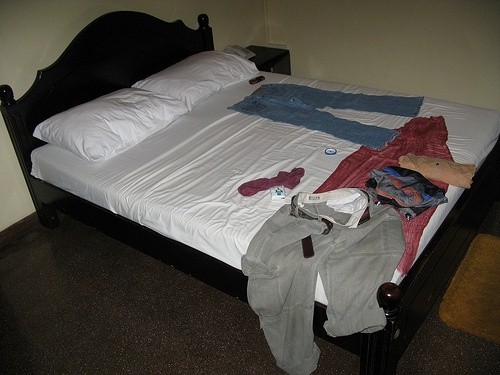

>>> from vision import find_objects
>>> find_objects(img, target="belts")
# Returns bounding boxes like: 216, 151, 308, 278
290, 187, 370, 258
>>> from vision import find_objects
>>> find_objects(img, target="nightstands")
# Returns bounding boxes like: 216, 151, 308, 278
244, 44, 292, 77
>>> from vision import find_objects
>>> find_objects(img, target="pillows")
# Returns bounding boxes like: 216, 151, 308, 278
32, 88, 191, 162
130, 50, 259, 113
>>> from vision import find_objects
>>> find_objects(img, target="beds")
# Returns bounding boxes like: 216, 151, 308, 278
0, 9, 500, 375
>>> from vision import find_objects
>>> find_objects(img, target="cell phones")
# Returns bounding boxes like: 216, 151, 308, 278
249, 75, 265, 84
